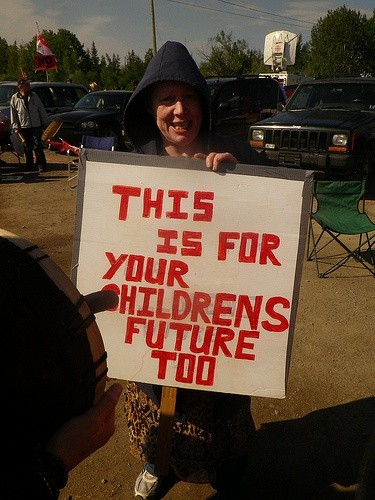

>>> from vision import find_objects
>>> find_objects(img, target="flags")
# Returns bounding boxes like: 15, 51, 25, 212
36, 30, 53, 55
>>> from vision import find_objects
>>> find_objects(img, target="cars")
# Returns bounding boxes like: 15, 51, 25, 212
46, 89, 138, 143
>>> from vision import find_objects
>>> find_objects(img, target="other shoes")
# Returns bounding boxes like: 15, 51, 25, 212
38, 163, 46, 171
135, 468, 161, 499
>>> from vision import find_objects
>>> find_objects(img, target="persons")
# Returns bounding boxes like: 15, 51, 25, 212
86, 82, 98, 103
121, 40, 259, 500
0, 290, 123, 500
10, 78, 50, 172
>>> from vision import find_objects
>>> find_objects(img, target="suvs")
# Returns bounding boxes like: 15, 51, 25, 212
246, 77, 375, 193
0, 82, 93, 138
198, 74, 286, 151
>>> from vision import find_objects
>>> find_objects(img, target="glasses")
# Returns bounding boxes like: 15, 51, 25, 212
159, 95, 199, 106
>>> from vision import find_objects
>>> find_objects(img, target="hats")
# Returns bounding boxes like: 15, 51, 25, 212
18, 77, 32, 84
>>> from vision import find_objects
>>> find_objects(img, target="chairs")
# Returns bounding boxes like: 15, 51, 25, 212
67, 136, 115, 186
307, 181, 375, 276
